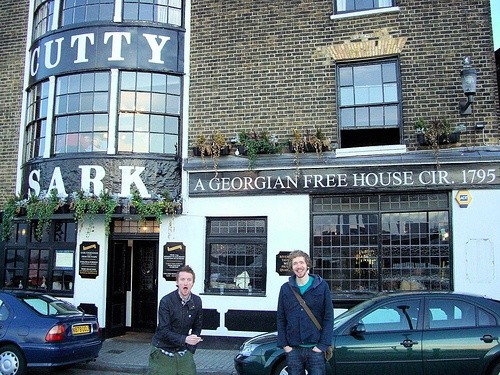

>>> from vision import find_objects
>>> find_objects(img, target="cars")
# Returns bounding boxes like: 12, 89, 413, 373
233, 290, 500, 375
0, 287, 105, 375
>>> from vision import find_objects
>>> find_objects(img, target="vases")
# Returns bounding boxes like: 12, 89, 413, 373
193, 146, 229, 156
416, 132, 460, 146
289, 142, 329, 153
237, 144, 267, 155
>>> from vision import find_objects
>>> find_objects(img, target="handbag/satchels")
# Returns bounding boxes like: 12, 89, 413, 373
324, 343, 335, 363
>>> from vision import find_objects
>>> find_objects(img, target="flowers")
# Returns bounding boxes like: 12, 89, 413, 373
1, 188, 182, 241
234, 131, 279, 173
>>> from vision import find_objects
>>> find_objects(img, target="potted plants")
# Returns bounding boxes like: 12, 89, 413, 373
192, 131, 229, 177
287, 128, 331, 172
413, 120, 463, 169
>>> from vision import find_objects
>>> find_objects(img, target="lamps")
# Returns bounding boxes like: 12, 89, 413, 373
459, 56, 477, 116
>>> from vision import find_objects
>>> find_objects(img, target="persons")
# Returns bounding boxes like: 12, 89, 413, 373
333, 281, 343, 290
79, 133, 132, 153
277, 249, 334, 375
148, 266, 203, 375
401, 268, 428, 291
